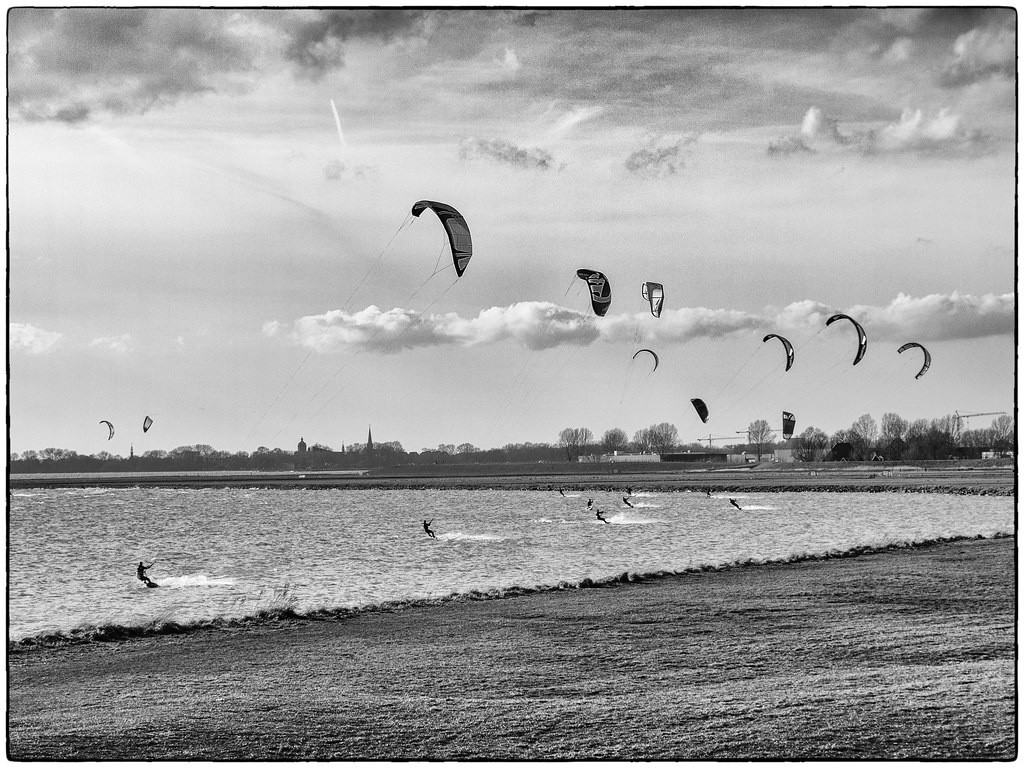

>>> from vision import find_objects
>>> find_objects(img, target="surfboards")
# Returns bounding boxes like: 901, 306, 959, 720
146, 582, 160, 589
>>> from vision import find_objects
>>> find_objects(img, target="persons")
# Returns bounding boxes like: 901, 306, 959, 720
707, 486, 712, 497
137, 562, 151, 584
588, 499, 593, 510
596, 509, 608, 523
627, 487, 633, 495
623, 497, 632, 507
730, 498, 741, 509
423, 520, 435, 538
560, 488, 564, 496
